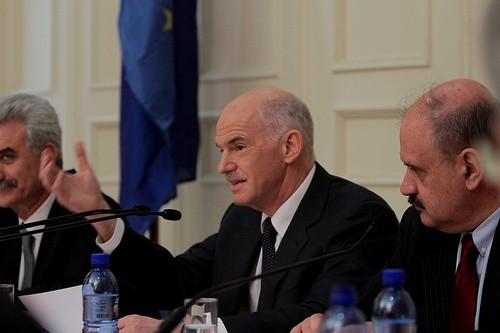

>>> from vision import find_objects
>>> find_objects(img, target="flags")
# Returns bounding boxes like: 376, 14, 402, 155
118, 0, 200, 236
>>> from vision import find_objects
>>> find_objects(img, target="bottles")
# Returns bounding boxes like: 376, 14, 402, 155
372, 269, 416, 333
82, 254, 120, 333
317, 285, 367, 333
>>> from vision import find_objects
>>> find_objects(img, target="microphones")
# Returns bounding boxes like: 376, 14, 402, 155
1, 205, 150, 233
154, 208, 385, 333
0, 209, 182, 242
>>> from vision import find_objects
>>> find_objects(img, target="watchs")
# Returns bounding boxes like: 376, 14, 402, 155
181, 324, 196, 333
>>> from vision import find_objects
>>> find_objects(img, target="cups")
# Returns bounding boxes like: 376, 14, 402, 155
184, 297, 218, 333
0, 284, 14, 303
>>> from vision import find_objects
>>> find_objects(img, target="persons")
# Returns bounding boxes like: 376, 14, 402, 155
38, 86, 402, 333
290, 78, 500, 333
0, 93, 128, 310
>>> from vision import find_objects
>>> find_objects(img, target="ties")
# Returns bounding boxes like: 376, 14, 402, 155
254, 217, 276, 290
447, 233, 479, 333
21, 222, 36, 294
